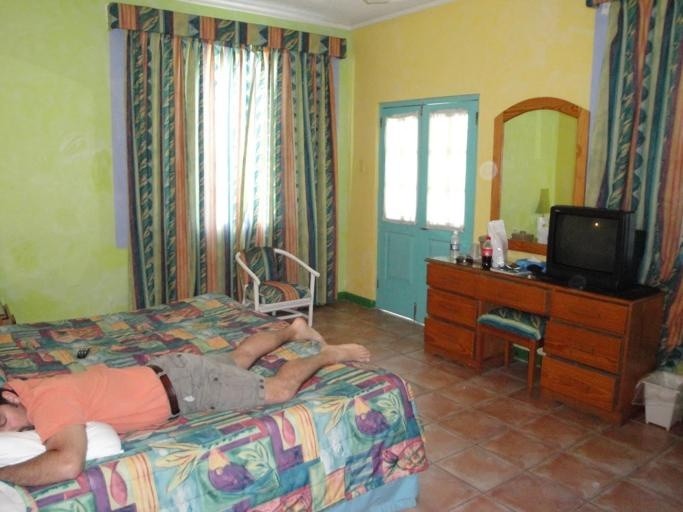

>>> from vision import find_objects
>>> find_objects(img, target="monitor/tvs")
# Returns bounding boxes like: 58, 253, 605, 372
548, 204, 648, 295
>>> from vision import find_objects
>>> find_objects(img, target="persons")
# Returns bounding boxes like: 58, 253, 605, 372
1, 316, 373, 488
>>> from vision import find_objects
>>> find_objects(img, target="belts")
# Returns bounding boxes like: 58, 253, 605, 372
147, 364, 181, 420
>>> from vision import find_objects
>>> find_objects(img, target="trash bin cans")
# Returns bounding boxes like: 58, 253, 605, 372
641, 370, 683, 432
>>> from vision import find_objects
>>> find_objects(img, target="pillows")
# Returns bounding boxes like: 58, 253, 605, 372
1, 422, 124, 468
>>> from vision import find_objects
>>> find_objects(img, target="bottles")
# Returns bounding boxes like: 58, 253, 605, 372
449, 230, 461, 262
481, 235, 494, 270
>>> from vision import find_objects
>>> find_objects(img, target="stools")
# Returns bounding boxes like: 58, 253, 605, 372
476, 307, 547, 396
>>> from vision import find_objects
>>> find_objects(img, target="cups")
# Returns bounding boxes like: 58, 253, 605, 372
469, 244, 480, 258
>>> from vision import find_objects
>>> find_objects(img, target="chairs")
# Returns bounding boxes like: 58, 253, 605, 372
235, 246, 321, 328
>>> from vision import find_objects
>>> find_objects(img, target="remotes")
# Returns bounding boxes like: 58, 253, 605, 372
77, 345, 91, 359
455, 254, 474, 265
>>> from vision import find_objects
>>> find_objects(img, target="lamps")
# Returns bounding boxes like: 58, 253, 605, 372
535, 188, 551, 244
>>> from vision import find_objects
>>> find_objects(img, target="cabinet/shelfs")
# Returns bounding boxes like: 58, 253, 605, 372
423, 251, 669, 426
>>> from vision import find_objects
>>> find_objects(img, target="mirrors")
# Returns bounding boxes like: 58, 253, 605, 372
489, 96, 591, 256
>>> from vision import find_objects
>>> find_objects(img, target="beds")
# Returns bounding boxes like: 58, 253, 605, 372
0, 292, 416, 512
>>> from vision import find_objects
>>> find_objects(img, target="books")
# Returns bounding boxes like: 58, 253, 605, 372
490, 264, 534, 275
